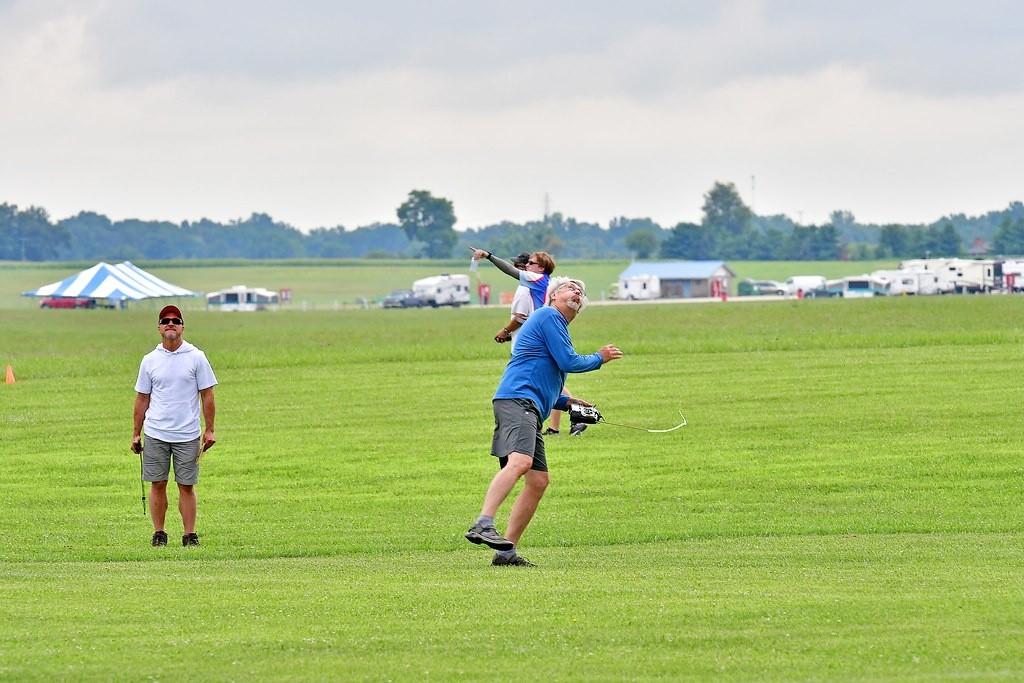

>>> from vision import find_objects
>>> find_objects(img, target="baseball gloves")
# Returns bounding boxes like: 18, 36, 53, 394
568, 404, 607, 436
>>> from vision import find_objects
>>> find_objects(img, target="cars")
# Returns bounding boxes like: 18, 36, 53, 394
804, 285, 843, 299
382, 288, 424, 309
754, 279, 787, 296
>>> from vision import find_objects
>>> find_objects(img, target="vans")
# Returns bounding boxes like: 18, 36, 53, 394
783, 276, 827, 295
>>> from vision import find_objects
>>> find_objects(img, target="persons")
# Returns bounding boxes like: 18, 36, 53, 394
464, 275, 624, 566
510, 253, 530, 271
469, 245, 588, 436
131, 305, 216, 547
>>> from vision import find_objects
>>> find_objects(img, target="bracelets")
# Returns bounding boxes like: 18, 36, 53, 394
504, 327, 510, 333
486, 251, 492, 259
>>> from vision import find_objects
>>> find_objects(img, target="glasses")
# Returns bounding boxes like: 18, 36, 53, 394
557, 283, 586, 297
525, 261, 539, 265
514, 263, 525, 268
159, 318, 182, 325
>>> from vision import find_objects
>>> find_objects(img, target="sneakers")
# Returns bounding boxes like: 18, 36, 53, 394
464, 523, 514, 551
181, 533, 201, 547
150, 531, 168, 547
569, 422, 587, 436
492, 553, 537, 567
541, 426, 559, 435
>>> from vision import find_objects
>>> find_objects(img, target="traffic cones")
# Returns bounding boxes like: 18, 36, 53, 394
4, 365, 17, 384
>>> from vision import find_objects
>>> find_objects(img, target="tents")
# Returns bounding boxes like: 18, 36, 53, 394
20, 261, 203, 312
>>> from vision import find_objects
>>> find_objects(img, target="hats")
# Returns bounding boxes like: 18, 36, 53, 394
510, 253, 530, 266
158, 305, 184, 325
